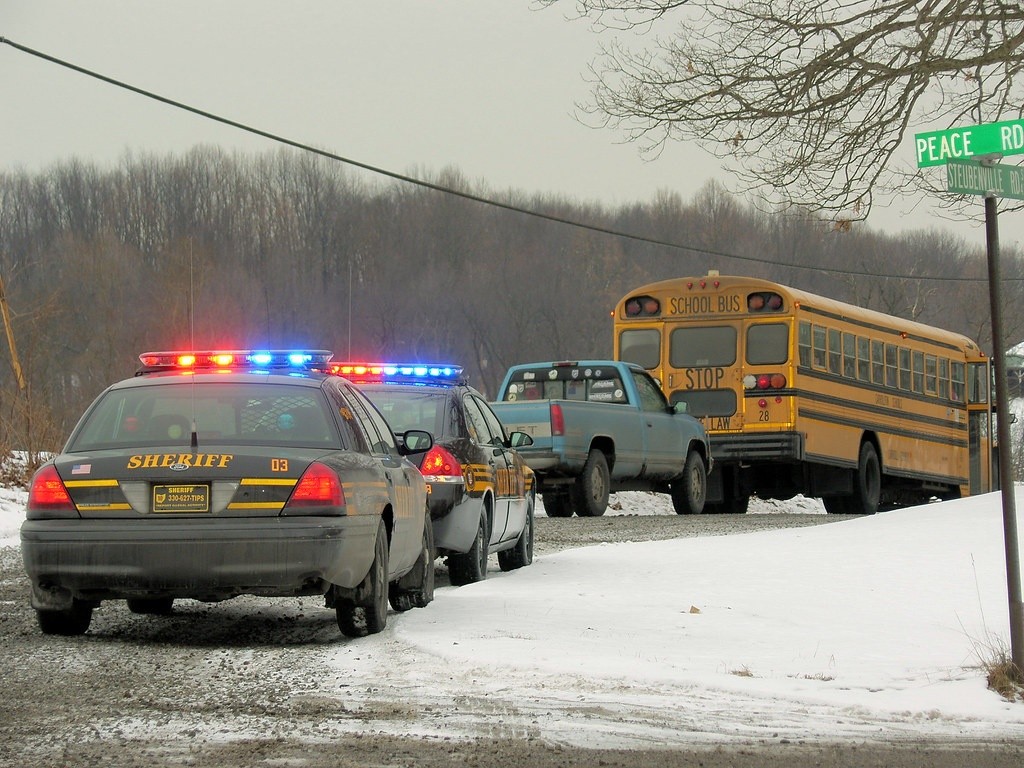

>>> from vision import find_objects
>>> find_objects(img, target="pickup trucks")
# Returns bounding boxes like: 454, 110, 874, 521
481, 357, 713, 516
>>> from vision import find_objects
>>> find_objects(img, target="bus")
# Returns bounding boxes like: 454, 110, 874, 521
611, 269, 995, 517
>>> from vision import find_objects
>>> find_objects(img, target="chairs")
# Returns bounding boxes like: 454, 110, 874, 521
149, 413, 191, 441
286, 407, 330, 442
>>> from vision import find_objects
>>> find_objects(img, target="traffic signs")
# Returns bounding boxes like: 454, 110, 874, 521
943, 157, 1024, 201
912, 115, 1024, 169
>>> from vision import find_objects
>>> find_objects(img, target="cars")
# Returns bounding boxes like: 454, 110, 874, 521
17, 346, 440, 639
310, 360, 538, 587
